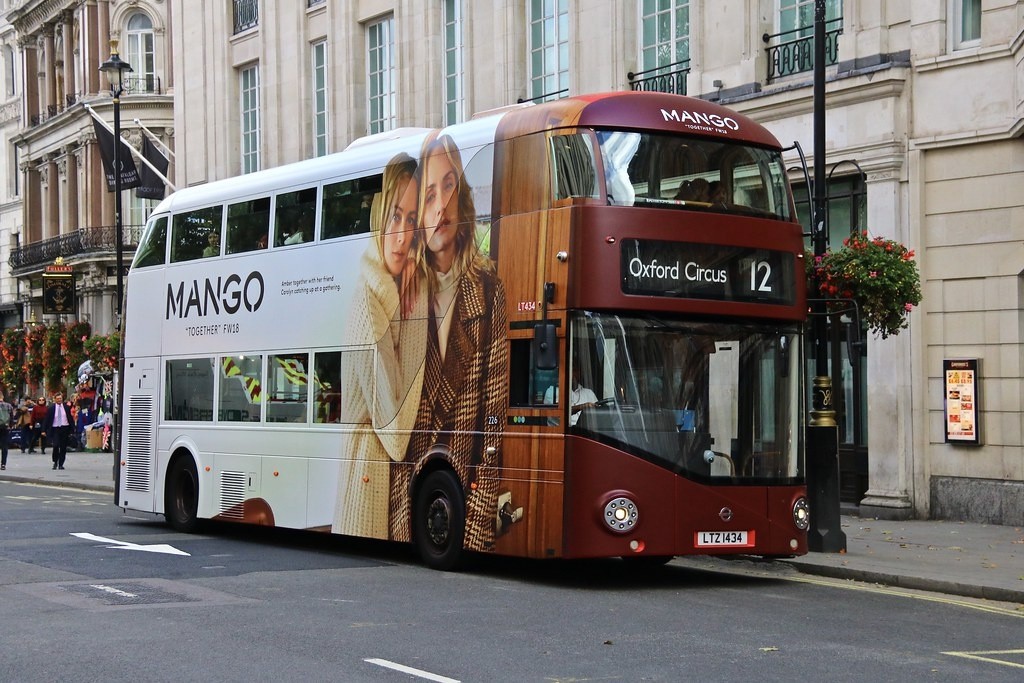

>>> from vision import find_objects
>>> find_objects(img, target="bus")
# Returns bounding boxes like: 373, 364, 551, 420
113, 91, 811, 571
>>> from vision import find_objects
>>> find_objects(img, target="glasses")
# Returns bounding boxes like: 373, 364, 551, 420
38, 399, 45, 401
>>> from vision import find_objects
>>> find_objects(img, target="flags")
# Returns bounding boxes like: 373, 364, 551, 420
91, 113, 142, 193
134, 132, 170, 200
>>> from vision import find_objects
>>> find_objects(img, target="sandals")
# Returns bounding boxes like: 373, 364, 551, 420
1, 464, 5, 470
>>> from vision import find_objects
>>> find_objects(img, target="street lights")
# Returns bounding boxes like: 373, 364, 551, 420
97, 39, 135, 329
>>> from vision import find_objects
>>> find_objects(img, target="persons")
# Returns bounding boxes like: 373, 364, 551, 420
329, 146, 432, 540
173, 190, 374, 423
40, 391, 77, 470
3, 395, 79, 455
543, 360, 599, 426
0, 390, 14, 470
385, 131, 509, 552
672, 178, 729, 205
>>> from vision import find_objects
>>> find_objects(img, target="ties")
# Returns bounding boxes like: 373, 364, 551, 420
58, 405, 62, 422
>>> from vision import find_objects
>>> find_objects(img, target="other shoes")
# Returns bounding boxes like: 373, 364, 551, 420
28, 450, 37, 454
21, 450, 25, 453
52, 461, 57, 470
41, 448, 46, 454
59, 464, 64, 469
